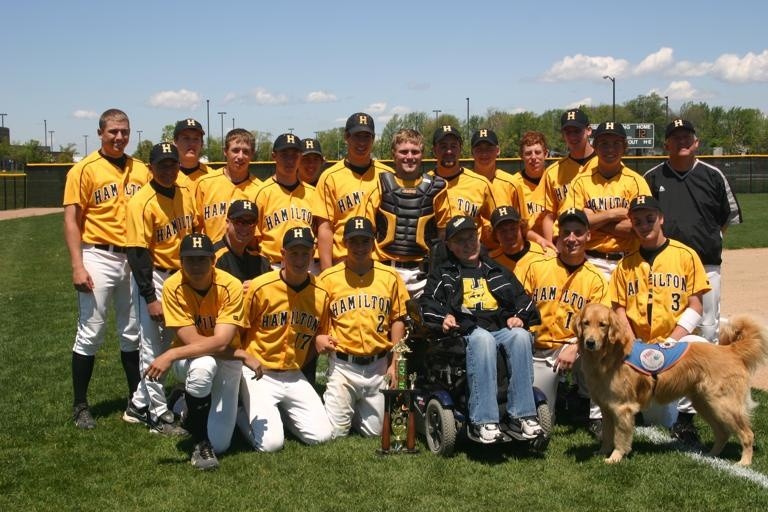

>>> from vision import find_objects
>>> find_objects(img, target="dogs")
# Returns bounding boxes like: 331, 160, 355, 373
572, 302, 768, 468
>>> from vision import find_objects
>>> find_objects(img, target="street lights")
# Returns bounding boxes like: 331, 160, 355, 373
136, 129, 144, 142
217, 111, 228, 147
663, 95, 669, 122
335, 128, 342, 161
415, 114, 420, 128
43, 119, 49, 147
313, 131, 319, 140
465, 97, 471, 141
288, 128, 295, 134
432, 109, 443, 128
47, 129, 55, 152
206, 99, 211, 145
0, 114, 9, 128
602, 75, 617, 124
82, 134, 89, 157
232, 116, 235, 130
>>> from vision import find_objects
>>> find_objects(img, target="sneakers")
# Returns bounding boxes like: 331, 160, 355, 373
672, 423, 708, 456
475, 414, 548, 444
72, 403, 98, 432
123, 403, 220, 473
588, 415, 605, 439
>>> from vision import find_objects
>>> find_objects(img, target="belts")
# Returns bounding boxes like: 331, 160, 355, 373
94, 245, 128, 254
334, 352, 386, 367
384, 260, 422, 269
154, 264, 178, 274
586, 250, 625, 261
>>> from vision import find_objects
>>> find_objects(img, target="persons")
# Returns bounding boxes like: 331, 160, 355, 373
610, 196, 712, 448
62, 109, 154, 431
122, 112, 651, 470
642, 120, 741, 337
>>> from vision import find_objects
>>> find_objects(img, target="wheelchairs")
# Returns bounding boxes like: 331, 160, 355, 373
405, 292, 554, 459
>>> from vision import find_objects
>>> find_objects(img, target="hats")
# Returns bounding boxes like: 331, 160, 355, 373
557, 109, 695, 227
431, 125, 520, 240
148, 112, 376, 257
